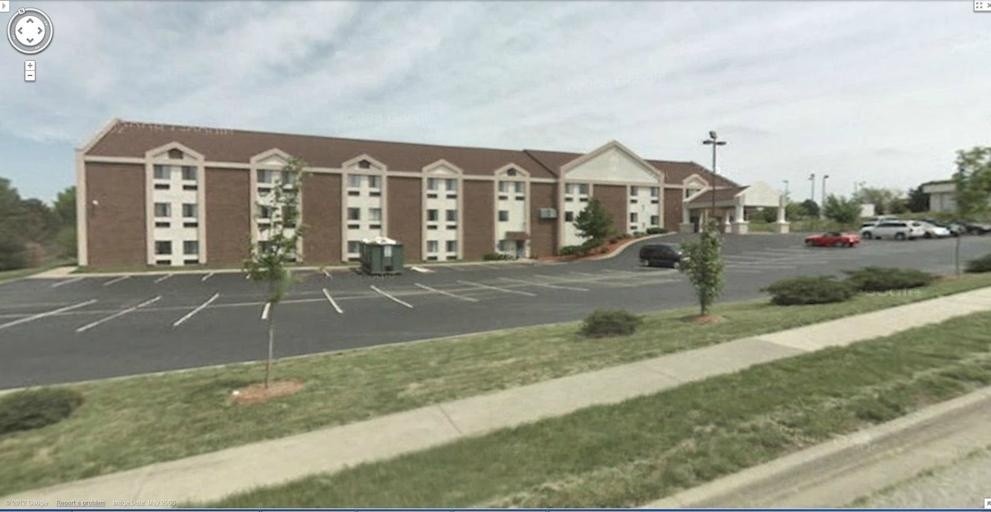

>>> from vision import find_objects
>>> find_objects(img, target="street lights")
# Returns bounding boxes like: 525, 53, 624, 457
822, 172, 829, 203
808, 171, 816, 201
703, 129, 727, 214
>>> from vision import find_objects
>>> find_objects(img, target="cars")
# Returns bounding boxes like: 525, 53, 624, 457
859, 215, 991, 241
802, 231, 860, 248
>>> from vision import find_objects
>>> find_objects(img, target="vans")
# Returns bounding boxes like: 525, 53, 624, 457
639, 243, 691, 269
